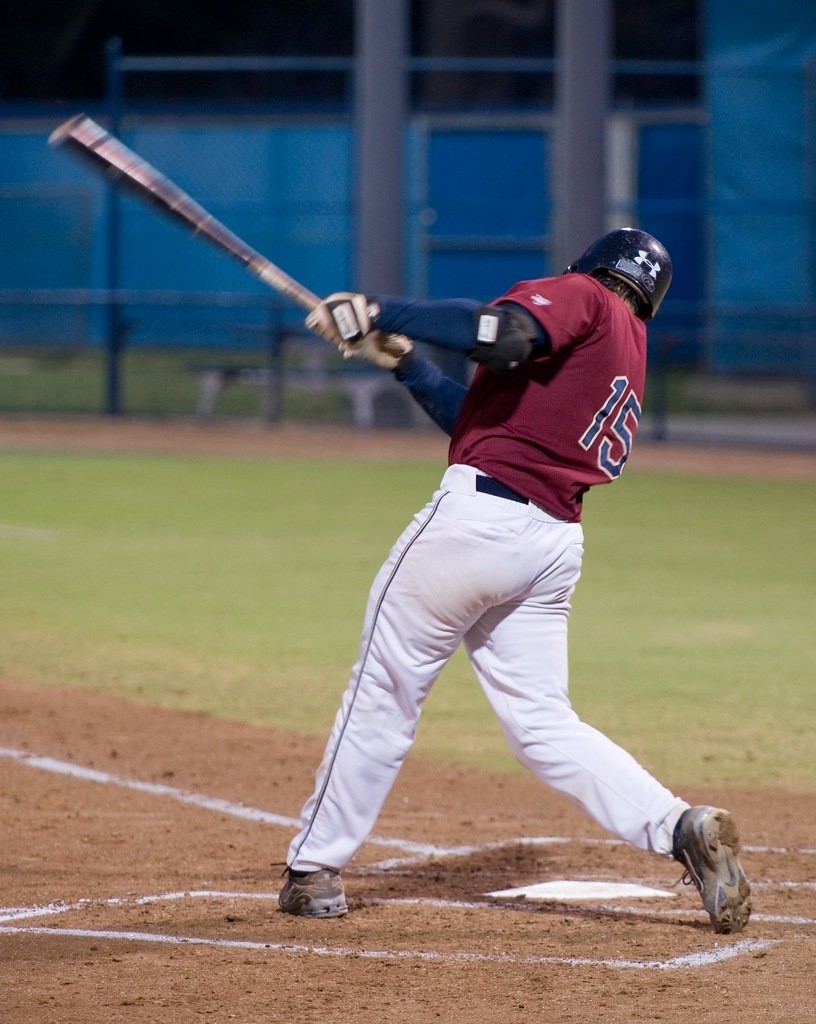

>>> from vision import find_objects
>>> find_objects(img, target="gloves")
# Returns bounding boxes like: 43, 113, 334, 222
342, 328, 413, 371
303, 291, 378, 351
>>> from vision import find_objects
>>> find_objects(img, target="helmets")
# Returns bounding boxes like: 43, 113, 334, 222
569, 228, 673, 320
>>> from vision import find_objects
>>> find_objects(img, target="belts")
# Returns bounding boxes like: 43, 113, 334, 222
475, 475, 530, 504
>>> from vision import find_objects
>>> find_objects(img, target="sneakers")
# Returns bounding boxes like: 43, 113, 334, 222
674, 804, 753, 935
270, 860, 349, 919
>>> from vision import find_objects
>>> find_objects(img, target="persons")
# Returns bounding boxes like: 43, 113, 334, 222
280, 228, 752, 935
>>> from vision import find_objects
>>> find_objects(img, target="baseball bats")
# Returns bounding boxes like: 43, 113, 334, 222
48, 114, 322, 313
